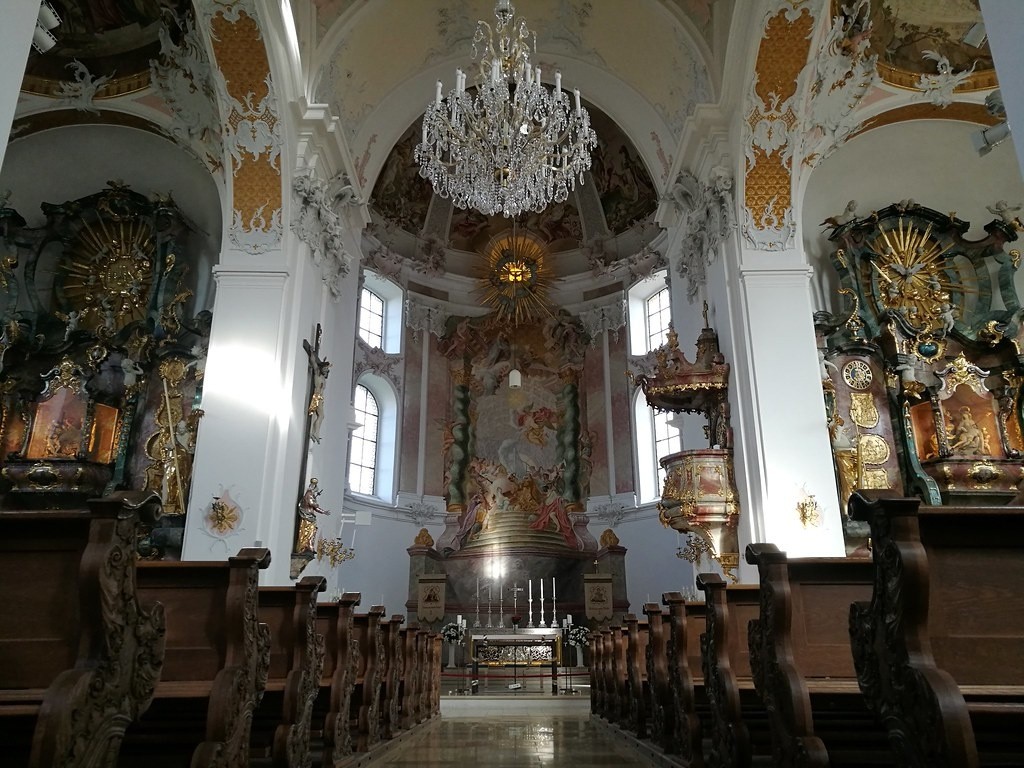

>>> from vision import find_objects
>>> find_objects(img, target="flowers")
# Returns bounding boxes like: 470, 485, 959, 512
568, 626, 589, 648
442, 623, 466, 647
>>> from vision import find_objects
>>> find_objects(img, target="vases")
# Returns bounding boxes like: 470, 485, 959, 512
447, 643, 457, 668
575, 645, 584, 667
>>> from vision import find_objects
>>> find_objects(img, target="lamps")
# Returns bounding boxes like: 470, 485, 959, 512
508, 219, 521, 389
209, 483, 238, 533
799, 482, 820, 525
414, 0, 598, 218
963, 23, 988, 50
970, 121, 1011, 158
31, 2, 63, 54
467, 218, 566, 329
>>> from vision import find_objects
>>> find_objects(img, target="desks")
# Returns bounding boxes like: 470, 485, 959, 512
468, 627, 562, 693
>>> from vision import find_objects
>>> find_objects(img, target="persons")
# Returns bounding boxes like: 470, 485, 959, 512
300, 323, 333, 446
928, 404, 993, 459
296, 478, 332, 555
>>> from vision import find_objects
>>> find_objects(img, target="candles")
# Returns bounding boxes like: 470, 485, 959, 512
351, 530, 356, 548
457, 615, 462, 623
541, 579, 543, 598
489, 584, 492, 597
339, 518, 345, 537
477, 578, 480, 597
499, 581, 502, 599
567, 614, 572, 624
529, 580, 532, 599
462, 620, 466, 627
563, 619, 567, 627
553, 577, 555, 598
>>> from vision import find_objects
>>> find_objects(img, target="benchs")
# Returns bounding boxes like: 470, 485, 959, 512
2, 492, 445, 768
585, 489, 1024, 768
